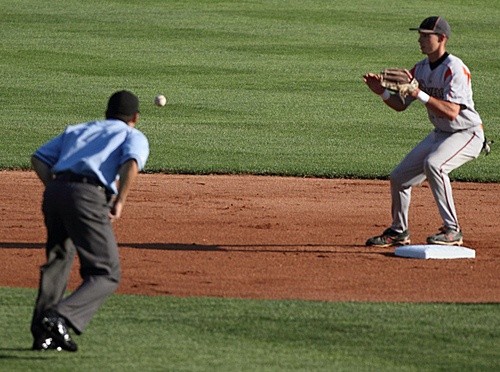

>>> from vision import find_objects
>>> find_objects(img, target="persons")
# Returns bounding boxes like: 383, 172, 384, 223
363, 16, 485, 245
31, 91, 150, 350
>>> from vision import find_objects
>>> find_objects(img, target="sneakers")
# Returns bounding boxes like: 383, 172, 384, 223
427, 225, 463, 246
41, 313, 78, 352
32, 335, 59, 351
367, 227, 410, 248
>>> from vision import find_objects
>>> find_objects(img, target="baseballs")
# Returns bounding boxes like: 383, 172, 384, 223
155, 95, 166, 107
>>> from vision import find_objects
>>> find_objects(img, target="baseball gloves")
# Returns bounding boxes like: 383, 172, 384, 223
380, 69, 418, 95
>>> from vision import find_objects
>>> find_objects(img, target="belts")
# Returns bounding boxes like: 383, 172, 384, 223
52, 174, 101, 185
435, 128, 467, 134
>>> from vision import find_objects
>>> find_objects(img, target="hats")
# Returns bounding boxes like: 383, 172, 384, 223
108, 90, 138, 115
409, 16, 451, 38
